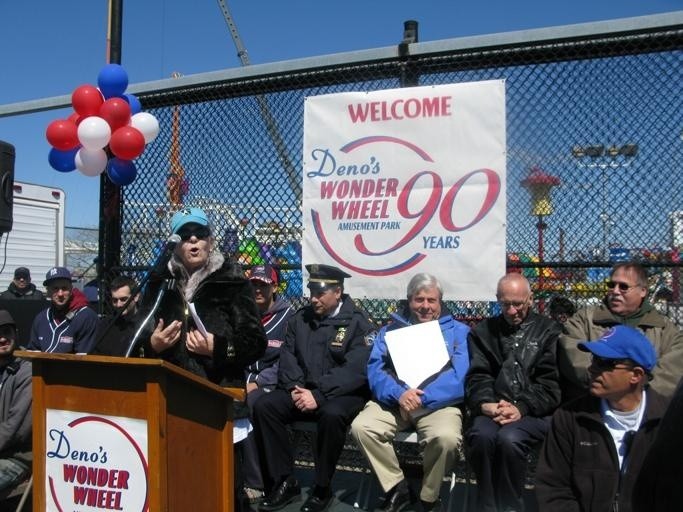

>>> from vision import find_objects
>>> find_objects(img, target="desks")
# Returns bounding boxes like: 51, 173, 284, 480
12, 347, 243, 512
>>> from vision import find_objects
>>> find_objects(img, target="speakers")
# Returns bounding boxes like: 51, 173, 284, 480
0, 140, 16, 235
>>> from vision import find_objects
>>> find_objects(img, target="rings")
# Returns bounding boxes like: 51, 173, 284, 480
169, 334, 174, 341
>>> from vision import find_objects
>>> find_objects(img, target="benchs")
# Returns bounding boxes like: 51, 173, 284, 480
291, 420, 470, 510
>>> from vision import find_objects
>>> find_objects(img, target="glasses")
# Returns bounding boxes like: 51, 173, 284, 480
176, 229, 209, 243
497, 294, 530, 309
606, 280, 643, 291
590, 355, 634, 372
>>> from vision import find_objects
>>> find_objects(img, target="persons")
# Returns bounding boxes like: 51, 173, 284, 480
95, 275, 154, 356
27, 267, 98, 354
0, 310, 32, 493
350, 275, 472, 512
465, 272, 563, 512
253, 264, 380, 512
558, 263, 683, 400
230, 265, 294, 504
0, 267, 46, 300
135, 207, 268, 512
536, 325, 683, 512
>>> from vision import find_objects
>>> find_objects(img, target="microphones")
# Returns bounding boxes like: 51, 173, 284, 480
152, 233, 183, 283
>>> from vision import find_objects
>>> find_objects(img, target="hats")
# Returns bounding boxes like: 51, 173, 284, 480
248, 265, 278, 286
304, 264, 352, 291
576, 325, 657, 373
0, 309, 15, 328
43, 266, 74, 287
14, 267, 31, 276
168, 208, 209, 238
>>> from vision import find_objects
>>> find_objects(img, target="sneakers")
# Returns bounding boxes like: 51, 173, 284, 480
239, 489, 265, 504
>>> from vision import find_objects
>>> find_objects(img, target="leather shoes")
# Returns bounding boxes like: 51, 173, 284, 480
406, 501, 446, 512
258, 480, 302, 512
373, 488, 417, 512
300, 483, 336, 512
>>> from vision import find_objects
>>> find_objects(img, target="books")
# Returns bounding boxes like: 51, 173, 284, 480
383, 320, 465, 419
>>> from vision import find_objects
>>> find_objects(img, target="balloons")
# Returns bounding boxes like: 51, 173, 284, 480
108, 126, 145, 160
101, 97, 132, 130
121, 94, 141, 115
46, 120, 80, 149
129, 112, 160, 144
77, 116, 112, 151
107, 160, 136, 185
97, 63, 130, 98
48, 146, 79, 172
73, 84, 104, 116
68, 111, 81, 123
75, 148, 108, 176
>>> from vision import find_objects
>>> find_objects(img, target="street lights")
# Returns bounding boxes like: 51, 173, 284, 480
521, 166, 560, 313
571, 143, 639, 261
